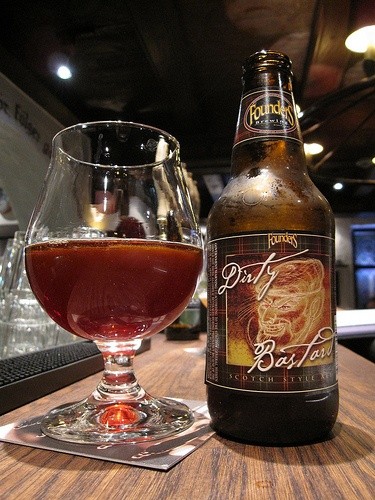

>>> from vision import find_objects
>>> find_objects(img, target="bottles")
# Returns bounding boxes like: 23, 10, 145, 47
207, 51, 340, 447
162, 210, 199, 341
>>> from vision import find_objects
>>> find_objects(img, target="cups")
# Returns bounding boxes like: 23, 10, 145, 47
0, 225, 74, 354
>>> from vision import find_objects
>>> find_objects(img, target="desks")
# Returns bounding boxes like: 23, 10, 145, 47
0, 333, 375, 500
336, 309, 375, 340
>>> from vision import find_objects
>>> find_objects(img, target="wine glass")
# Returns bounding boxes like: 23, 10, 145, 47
26, 122, 204, 445
